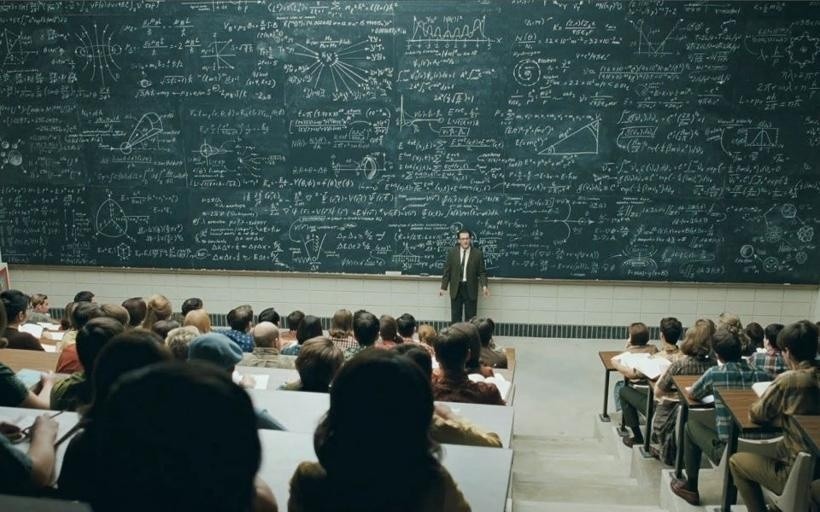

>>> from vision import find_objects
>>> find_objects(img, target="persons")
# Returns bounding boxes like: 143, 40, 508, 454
607, 312, 820, 512
1, 286, 508, 512
438, 229, 491, 326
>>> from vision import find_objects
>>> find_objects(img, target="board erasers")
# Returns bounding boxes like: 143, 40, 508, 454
420, 272, 429, 276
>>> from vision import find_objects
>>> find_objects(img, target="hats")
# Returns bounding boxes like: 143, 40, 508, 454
190, 332, 243, 367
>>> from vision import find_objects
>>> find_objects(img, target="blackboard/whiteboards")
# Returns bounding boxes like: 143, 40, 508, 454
0, 0, 820, 285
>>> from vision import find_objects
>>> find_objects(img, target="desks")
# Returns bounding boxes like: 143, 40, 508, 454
0, 320, 516, 512
599, 350, 820, 511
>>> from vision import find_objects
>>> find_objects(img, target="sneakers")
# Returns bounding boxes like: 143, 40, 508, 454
623, 436, 636, 446
671, 479, 699, 505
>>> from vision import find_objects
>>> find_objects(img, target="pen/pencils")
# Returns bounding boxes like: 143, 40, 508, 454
21, 408, 66, 431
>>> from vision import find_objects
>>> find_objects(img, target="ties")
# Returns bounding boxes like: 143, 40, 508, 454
460, 250, 466, 276
